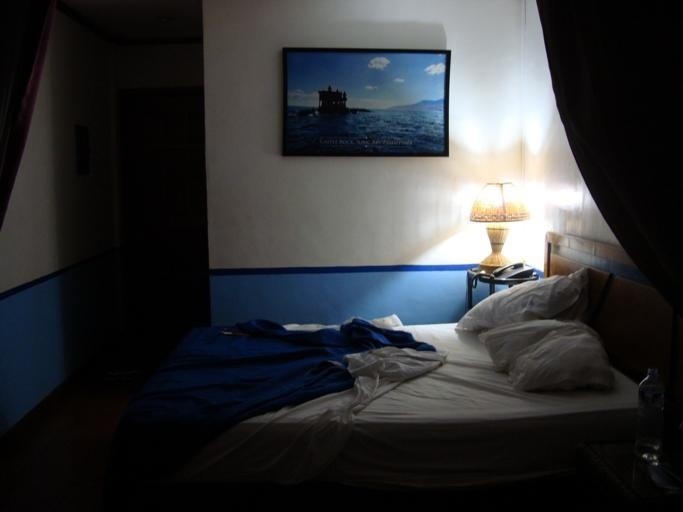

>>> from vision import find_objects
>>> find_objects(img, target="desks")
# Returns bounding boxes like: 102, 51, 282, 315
467, 270, 538, 316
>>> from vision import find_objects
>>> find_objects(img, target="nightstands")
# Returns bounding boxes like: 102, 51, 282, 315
573, 439, 683, 512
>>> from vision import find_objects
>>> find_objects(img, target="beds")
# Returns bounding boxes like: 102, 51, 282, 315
105, 232, 682, 511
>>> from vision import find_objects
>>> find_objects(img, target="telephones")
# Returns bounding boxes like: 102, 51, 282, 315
493, 262, 534, 278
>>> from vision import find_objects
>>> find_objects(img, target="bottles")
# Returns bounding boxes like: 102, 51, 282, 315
637, 368, 664, 466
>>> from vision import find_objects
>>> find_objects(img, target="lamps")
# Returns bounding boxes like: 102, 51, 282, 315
469, 181, 530, 273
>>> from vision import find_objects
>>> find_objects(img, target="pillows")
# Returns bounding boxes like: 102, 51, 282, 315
477, 320, 608, 392
455, 267, 593, 331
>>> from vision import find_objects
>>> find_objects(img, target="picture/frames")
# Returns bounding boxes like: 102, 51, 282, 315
281, 47, 452, 158
